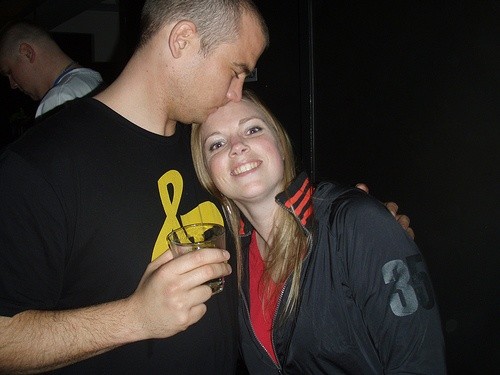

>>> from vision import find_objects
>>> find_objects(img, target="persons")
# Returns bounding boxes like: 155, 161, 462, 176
0, 1, 417, 375
189, 90, 448, 375
0, 18, 104, 119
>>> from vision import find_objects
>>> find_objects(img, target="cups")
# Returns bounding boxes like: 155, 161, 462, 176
166, 223, 228, 297
